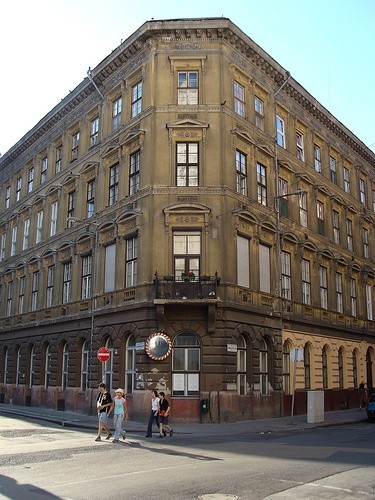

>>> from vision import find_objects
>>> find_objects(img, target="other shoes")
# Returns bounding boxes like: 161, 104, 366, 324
144, 434, 152, 437
163, 430, 167, 436
170, 429, 173, 436
156, 435, 163, 438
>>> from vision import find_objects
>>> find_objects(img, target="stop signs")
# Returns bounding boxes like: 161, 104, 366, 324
97, 347, 110, 362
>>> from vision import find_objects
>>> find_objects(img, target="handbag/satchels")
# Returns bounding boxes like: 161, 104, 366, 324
161, 410, 170, 416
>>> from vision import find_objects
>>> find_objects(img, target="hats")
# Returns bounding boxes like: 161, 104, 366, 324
97, 383, 106, 387
114, 388, 124, 395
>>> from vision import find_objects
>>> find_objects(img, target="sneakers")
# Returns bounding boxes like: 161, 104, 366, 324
105, 433, 112, 439
123, 431, 126, 440
94, 436, 101, 441
111, 439, 119, 443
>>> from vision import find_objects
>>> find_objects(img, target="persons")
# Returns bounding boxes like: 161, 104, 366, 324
156, 391, 173, 439
146, 390, 167, 438
93, 382, 112, 441
107, 388, 129, 443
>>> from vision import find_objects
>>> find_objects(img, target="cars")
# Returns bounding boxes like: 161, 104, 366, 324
366, 393, 375, 418
